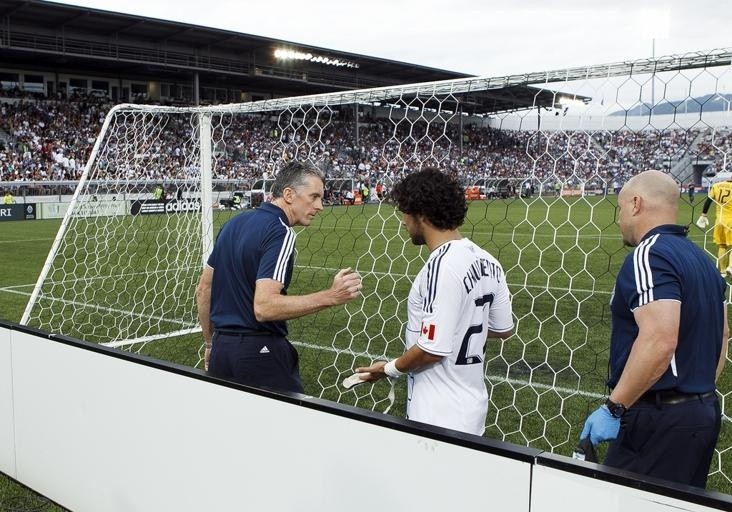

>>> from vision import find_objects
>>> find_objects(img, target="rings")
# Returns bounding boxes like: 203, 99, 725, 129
355, 285, 360, 292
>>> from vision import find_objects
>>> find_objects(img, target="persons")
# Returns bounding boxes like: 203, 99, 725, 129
192, 158, 366, 393
569, 166, 727, 490
697, 177, 732, 278
352, 165, 517, 438
1, 77, 732, 206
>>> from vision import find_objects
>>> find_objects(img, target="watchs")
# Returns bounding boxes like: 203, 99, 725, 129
602, 396, 628, 421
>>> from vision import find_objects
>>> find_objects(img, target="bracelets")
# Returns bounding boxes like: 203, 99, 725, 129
383, 359, 407, 380
205, 342, 213, 350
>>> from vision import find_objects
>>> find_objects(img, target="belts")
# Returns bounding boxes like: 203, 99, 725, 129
646, 392, 712, 405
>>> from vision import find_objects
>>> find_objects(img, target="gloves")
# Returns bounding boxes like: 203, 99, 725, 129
695, 215, 710, 229
580, 403, 622, 446
